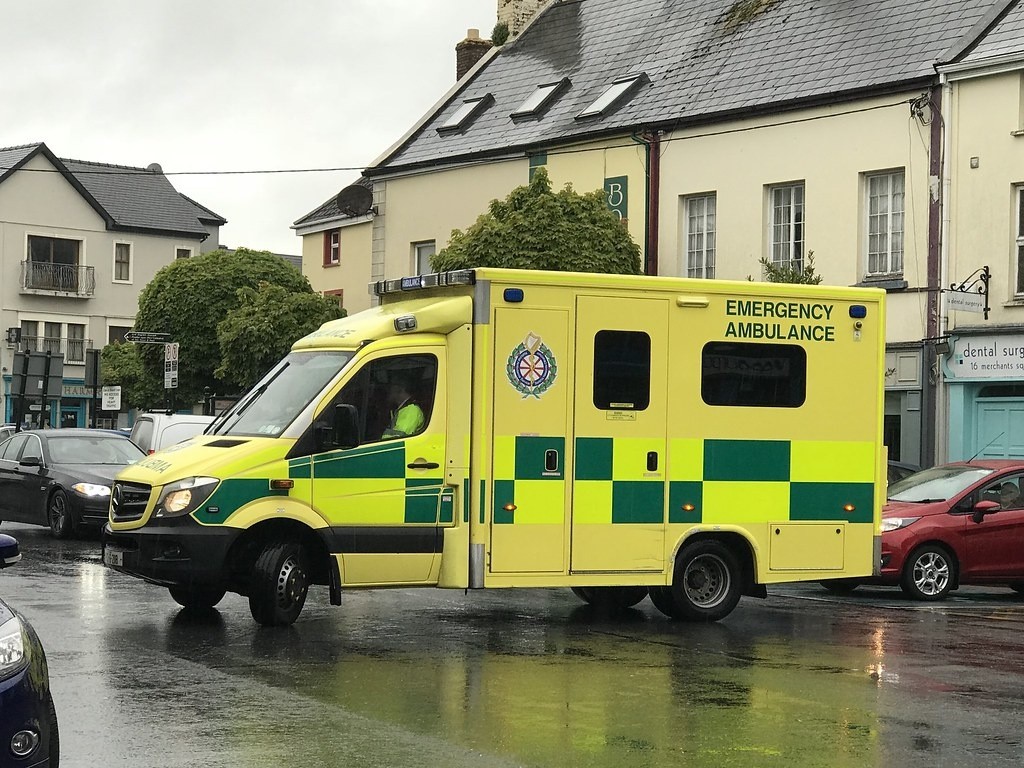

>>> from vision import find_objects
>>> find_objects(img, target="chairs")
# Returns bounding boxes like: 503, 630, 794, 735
983, 482, 1002, 503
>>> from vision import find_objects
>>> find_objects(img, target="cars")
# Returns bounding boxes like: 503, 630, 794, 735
1, 535, 61, 767
0, 428, 150, 538
819, 459, 1024, 601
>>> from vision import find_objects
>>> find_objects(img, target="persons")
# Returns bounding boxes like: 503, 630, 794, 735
991, 481, 1024, 512
378, 372, 426, 441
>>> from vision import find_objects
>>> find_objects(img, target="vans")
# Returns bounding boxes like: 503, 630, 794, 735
131, 409, 273, 459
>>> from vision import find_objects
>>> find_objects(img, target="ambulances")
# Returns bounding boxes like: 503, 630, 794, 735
101, 266, 889, 620
